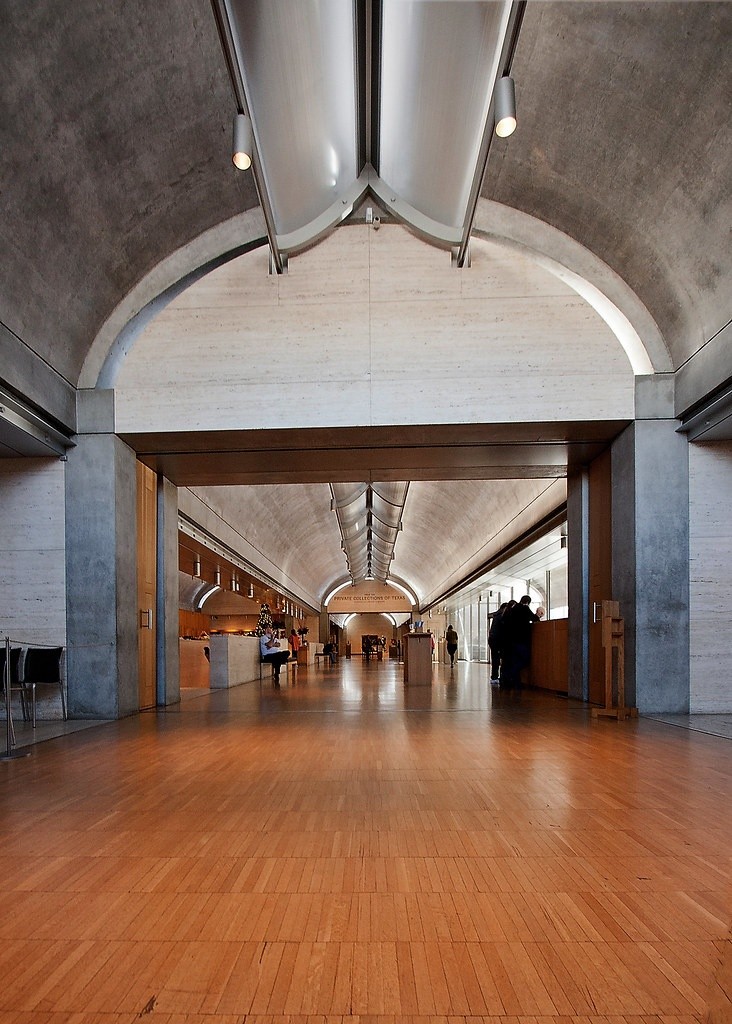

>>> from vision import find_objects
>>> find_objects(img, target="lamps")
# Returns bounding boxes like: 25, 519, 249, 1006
341, 539, 345, 548
232, 110, 253, 171
490, 591, 493, 596
479, 596, 482, 601
429, 607, 446, 618
560, 537, 566, 549
391, 552, 395, 560
331, 497, 337, 511
193, 562, 303, 620
494, 76, 517, 137
398, 521, 403, 532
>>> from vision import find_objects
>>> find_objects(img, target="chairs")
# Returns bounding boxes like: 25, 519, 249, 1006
0, 646, 66, 745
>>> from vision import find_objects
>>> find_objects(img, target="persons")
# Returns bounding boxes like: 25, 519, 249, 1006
323, 641, 337, 663
536, 606, 547, 621
487, 595, 540, 688
260, 623, 290, 680
427, 629, 435, 654
446, 624, 458, 669
364, 634, 373, 662
288, 629, 299, 665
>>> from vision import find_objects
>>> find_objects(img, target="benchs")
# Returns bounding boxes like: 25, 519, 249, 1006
314, 652, 339, 664
259, 658, 298, 679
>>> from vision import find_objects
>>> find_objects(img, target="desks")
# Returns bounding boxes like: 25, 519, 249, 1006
403, 633, 433, 687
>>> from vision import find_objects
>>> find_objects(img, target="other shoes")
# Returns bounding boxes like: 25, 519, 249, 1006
491, 680, 499, 684
451, 663, 454, 668
